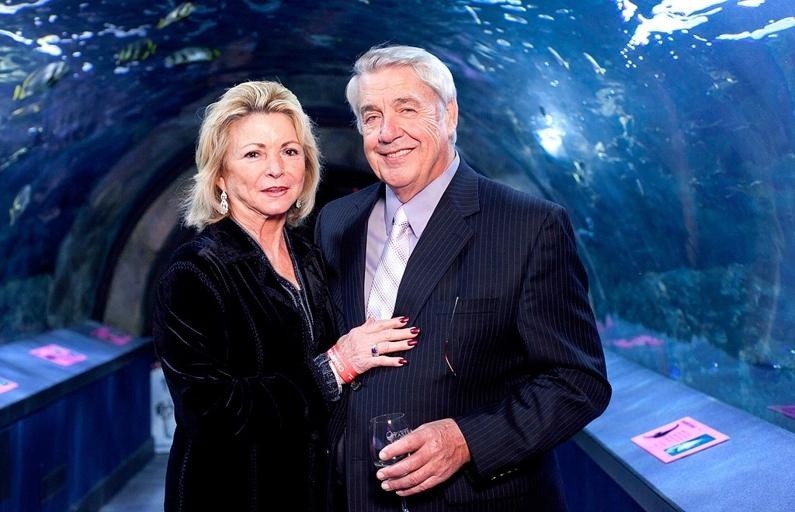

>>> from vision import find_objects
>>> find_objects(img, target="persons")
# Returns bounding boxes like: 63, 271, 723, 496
147, 78, 422, 512
310, 41, 613, 510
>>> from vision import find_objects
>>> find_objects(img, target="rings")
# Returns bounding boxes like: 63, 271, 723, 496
371, 343, 380, 356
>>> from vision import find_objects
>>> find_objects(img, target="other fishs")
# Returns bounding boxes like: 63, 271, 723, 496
533, 93, 638, 193
1, 1, 224, 231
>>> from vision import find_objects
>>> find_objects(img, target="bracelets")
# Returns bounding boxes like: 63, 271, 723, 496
325, 345, 359, 390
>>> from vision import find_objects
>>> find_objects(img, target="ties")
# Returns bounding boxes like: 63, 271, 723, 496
365, 206, 410, 322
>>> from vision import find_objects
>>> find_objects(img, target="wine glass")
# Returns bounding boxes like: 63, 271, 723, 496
368, 414, 416, 511
